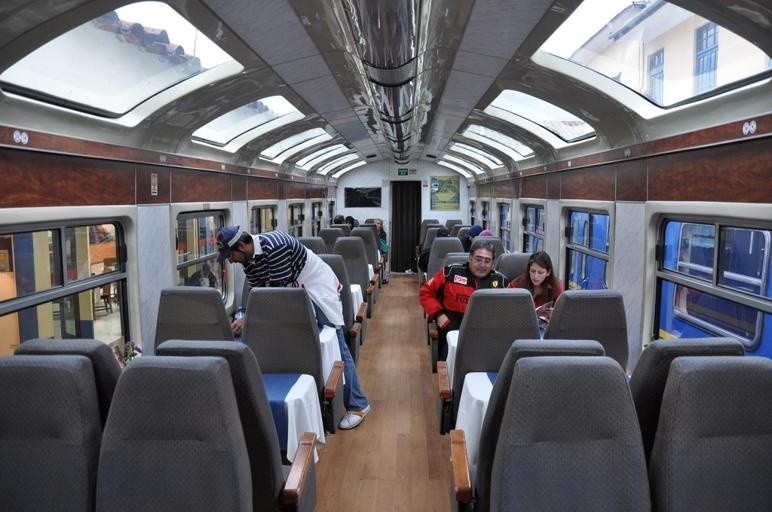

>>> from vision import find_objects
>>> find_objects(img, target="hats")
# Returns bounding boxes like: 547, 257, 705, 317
479, 230, 492, 235
468, 225, 481, 237
216, 224, 242, 263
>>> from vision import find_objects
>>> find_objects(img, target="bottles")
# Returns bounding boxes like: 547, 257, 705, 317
124, 342, 136, 365
234, 307, 245, 322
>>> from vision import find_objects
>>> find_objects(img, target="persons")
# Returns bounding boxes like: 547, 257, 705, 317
506, 251, 564, 324
418, 239, 509, 361
215, 226, 372, 430
334, 214, 493, 273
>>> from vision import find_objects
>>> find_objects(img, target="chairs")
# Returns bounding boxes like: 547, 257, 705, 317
436, 289, 771, 511
239, 218, 387, 365
416, 215, 546, 369
2, 288, 345, 509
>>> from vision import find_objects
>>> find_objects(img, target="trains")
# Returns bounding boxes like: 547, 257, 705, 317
482, 203, 772, 365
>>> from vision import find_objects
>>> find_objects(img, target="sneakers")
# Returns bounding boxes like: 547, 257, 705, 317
405, 268, 417, 275
338, 404, 371, 430
